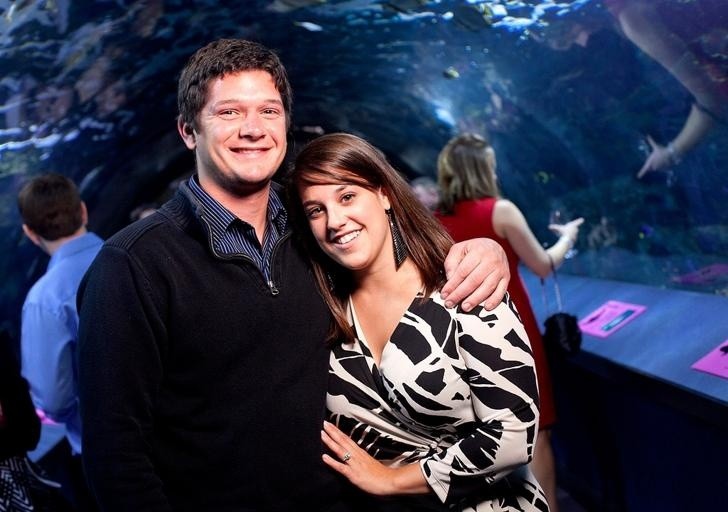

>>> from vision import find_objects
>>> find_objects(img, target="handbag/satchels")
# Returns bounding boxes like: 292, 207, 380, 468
540, 310, 582, 358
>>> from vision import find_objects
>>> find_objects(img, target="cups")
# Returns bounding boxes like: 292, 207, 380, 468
549, 211, 572, 239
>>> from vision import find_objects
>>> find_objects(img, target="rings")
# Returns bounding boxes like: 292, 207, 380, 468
342, 453, 351, 459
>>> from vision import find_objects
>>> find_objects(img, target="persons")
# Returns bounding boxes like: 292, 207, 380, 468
17, 173, 104, 510
76, 36, 511, 512
280, 131, 550, 512
527, 1, 728, 181
410, 172, 443, 215
422, 131, 585, 511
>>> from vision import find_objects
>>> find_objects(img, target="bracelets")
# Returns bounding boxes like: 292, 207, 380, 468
668, 143, 682, 167
559, 236, 576, 250
